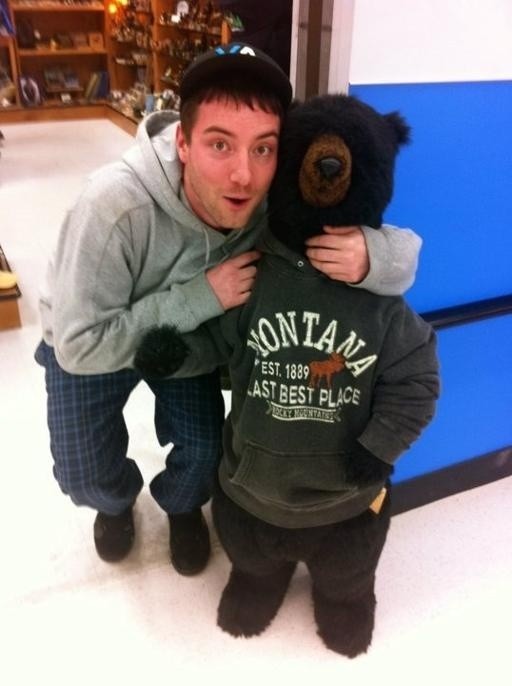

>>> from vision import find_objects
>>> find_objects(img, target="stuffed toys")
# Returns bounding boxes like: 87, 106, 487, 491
134, 93, 439, 660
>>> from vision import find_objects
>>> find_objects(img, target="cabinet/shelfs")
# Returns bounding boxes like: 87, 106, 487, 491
0, 0, 293, 138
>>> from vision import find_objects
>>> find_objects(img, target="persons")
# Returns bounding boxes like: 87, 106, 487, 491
34, 41, 422, 577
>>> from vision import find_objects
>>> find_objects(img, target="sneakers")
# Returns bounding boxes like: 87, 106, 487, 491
95, 508, 136, 563
166, 508, 210, 577
